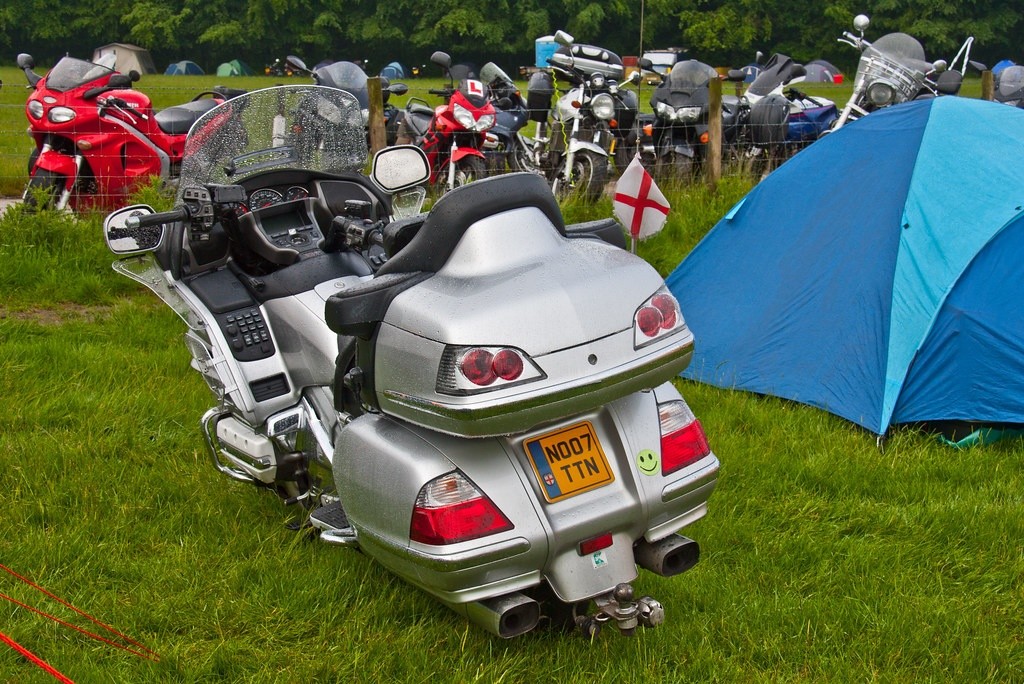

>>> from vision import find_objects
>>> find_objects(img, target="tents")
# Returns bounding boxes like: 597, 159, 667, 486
216, 59, 256, 78
164, 60, 205, 76
446, 64, 474, 81
664, 95, 1024, 436
803, 60, 840, 83
990, 60, 1015, 74
740, 64, 763, 83
378, 61, 408, 80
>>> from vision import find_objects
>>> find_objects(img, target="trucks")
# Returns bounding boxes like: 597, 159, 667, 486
642, 53, 678, 86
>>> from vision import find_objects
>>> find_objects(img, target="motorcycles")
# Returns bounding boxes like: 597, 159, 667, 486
411, 30, 840, 207
15, 54, 249, 222
102, 84, 722, 642
968, 59, 1024, 111
820, 13, 974, 149
264, 54, 431, 163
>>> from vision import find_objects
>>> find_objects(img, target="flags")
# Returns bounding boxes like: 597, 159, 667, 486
613, 158, 671, 240
